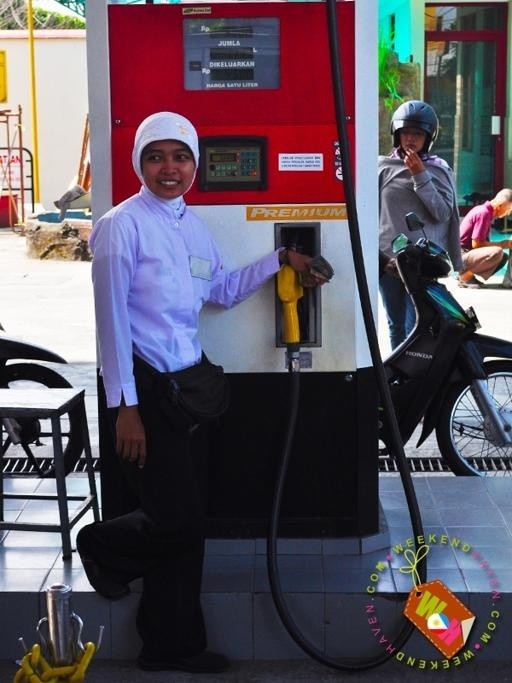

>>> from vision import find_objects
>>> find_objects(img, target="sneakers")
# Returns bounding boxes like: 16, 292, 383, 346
458, 276, 487, 288
137, 641, 233, 674
77, 524, 131, 601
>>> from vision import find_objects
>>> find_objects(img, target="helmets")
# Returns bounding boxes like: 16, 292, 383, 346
390, 99, 440, 152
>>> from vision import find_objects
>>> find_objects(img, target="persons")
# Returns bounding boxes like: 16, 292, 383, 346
85, 108, 331, 674
378, 99, 467, 424
456, 187, 512, 290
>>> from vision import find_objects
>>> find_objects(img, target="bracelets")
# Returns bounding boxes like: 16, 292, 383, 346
283, 250, 290, 264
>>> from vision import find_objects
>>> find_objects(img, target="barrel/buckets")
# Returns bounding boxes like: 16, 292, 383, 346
0, 193, 19, 230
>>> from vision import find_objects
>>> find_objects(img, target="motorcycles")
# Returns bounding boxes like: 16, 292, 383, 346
377, 212, 512, 477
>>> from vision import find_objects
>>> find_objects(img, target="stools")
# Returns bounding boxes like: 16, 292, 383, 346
0, 389, 101, 559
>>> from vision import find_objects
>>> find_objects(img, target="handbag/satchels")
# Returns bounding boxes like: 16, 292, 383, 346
156, 358, 237, 433
